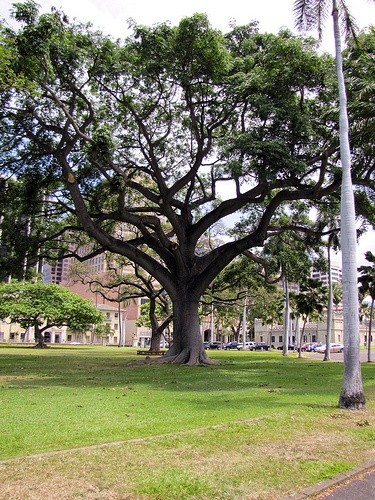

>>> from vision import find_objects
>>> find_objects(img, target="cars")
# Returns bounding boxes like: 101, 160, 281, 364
223, 341, 239, 350
237, 342, 255, 350
277, 345, 295, 350
315, 343, 343, 353
296, 343, 314, 352
137, 340, 224, 351
307, 343, 322, 352
249, 342, 273, 351
